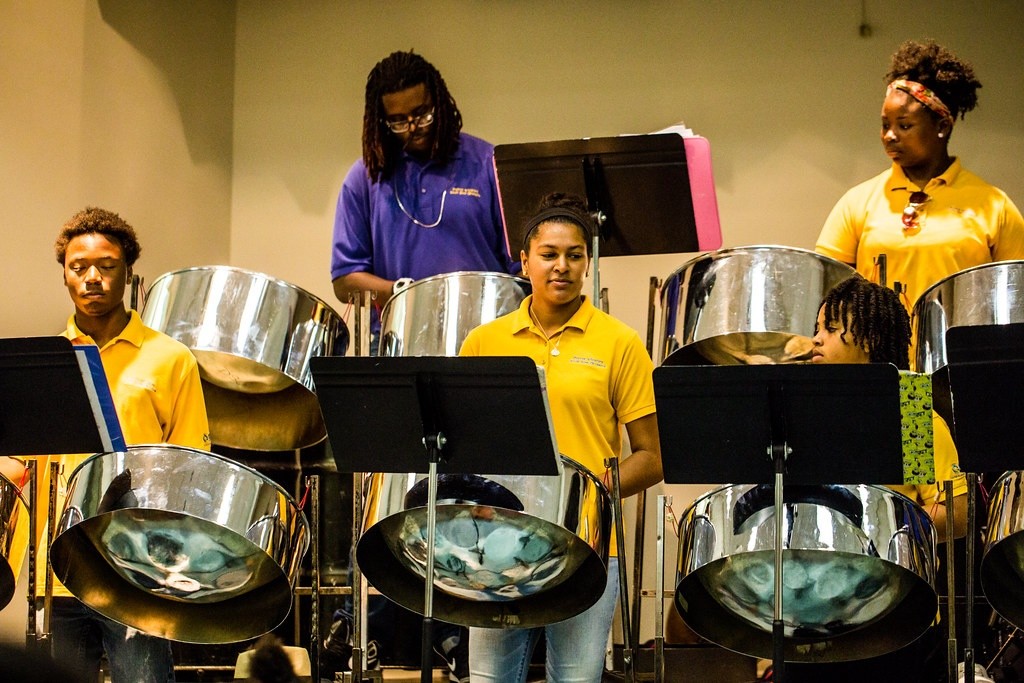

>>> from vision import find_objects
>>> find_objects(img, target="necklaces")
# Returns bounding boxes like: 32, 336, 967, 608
391, 177, 447, 228
529, 303, 567, 356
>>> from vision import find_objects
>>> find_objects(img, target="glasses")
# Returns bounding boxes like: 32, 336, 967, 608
386, 106, 437, 133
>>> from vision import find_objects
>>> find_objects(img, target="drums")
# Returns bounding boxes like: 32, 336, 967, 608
140, 265, 349, 452
357, 447, 614, 630
0, 471, 31, 611
906, 258, 1024, 417
46, 444, 311, 645
979, 468, 1024, 630
656, 244, 867, 365
377, 268, 532, 356
675, 476, 938, 666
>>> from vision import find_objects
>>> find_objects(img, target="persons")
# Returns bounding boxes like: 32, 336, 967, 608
810, 277, 972, 546
814, 41, 1024, 373
457, 189, 663, 683
313, 49, 532, 650
2, 204, 212, 682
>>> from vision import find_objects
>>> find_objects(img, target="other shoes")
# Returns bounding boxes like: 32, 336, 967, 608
320, 618, 354, 661
958, 662, 996, 683
